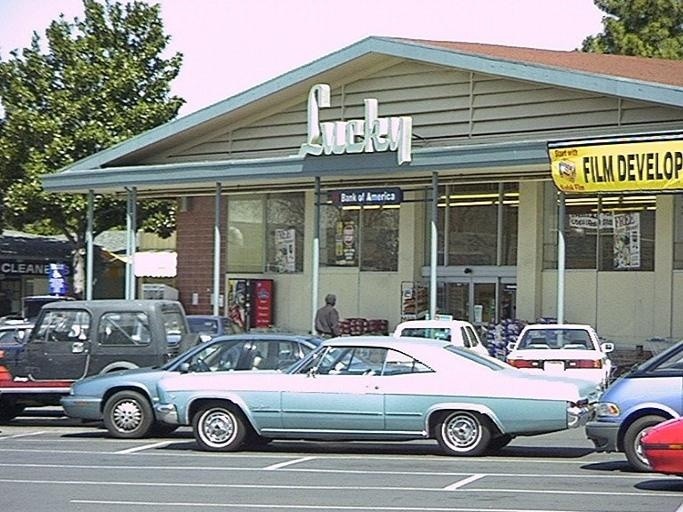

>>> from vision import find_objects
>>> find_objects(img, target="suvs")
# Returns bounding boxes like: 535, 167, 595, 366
0, 295, 202, 425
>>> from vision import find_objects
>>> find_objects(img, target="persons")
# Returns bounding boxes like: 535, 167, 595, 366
315, 295, 340, 338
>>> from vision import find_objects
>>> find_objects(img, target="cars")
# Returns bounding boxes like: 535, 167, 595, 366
641, 416, 683, 475
389, 319, 489, 356
154, 333, 598, 455
185, 315, 240, 339
61, 333, 412, 438
506, 324, 614, 397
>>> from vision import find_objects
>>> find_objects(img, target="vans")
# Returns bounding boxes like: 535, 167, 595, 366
583, 341, 682, 470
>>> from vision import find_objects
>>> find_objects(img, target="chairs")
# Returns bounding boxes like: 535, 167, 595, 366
570, 340, 587, 349
529, 338, 547, 349
106, 312, 135, 344
234, 344, 253, 370
257, 343, 280, 369
